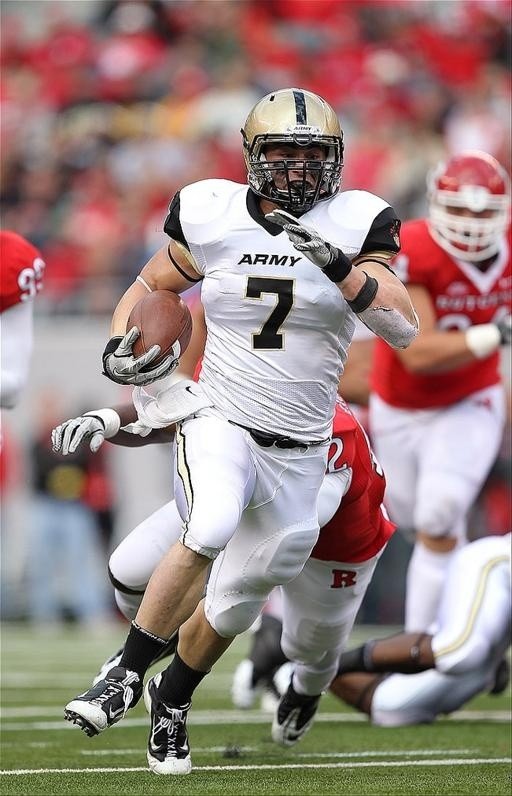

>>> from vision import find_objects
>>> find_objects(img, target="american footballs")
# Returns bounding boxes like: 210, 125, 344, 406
126, 289, 191, 371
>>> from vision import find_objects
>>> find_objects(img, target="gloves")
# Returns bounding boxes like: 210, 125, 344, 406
48, 405, 122, 455
460, 304, 512, 356
97, 326, 182, 389
258, 206, 351, 284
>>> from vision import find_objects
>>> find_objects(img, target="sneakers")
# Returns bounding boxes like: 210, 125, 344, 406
269, 674, 326, 750
65, 667, 145, 739
94, 624, 178, 688
228, 656, 268, 708
141, 668, 194, 779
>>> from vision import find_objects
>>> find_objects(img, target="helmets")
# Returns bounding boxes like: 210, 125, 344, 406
427, 151, 511, 263
241, 84, 346, 212
